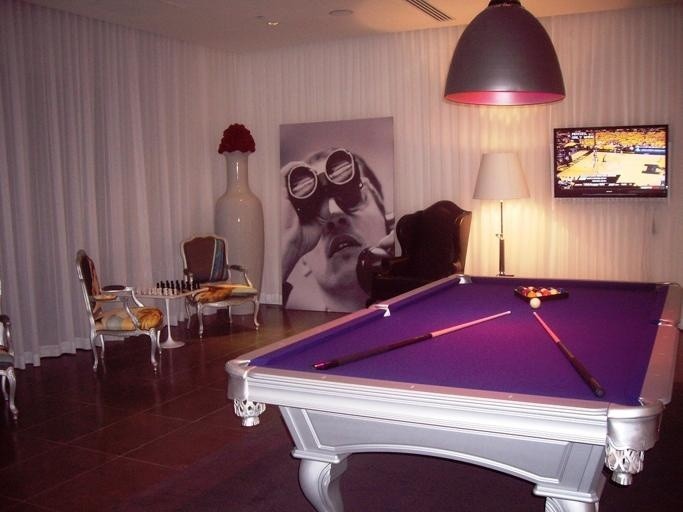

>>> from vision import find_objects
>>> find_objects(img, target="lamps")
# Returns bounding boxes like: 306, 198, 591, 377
472, 150, 532, 278
443, 1, 567, 109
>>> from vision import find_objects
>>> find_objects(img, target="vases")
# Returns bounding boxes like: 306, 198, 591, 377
214, 153, 264, 315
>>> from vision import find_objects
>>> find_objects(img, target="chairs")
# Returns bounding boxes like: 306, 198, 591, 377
72, 233, 261, 373
0, 309, 17, 417
356, 200, 471, 307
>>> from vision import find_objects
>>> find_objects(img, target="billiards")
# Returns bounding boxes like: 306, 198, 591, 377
517, 285, 565, 298
530, 298, 541, 309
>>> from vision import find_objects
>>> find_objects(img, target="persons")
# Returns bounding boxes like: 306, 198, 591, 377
282, 145, 395, 313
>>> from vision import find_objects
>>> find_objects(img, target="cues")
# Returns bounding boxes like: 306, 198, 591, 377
312, 310, 512, 371
532, 312, 605, 398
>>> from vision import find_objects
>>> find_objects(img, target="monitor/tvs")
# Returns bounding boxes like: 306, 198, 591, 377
554, 124, 668, 198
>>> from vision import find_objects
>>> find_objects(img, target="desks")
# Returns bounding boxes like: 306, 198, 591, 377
222, 272, 682, 511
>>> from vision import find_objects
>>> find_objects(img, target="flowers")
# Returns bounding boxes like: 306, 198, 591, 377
216, 122, 256, 153
